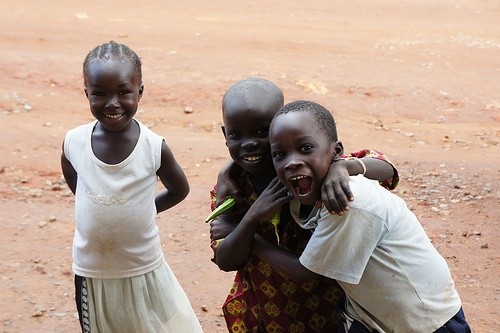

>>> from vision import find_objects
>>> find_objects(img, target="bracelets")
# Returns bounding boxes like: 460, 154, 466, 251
349, 157, 367, 175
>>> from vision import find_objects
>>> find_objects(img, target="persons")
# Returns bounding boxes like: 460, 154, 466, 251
60, 41, 203, 333
209, 101, 474, 333
209, 78, 399, 333
